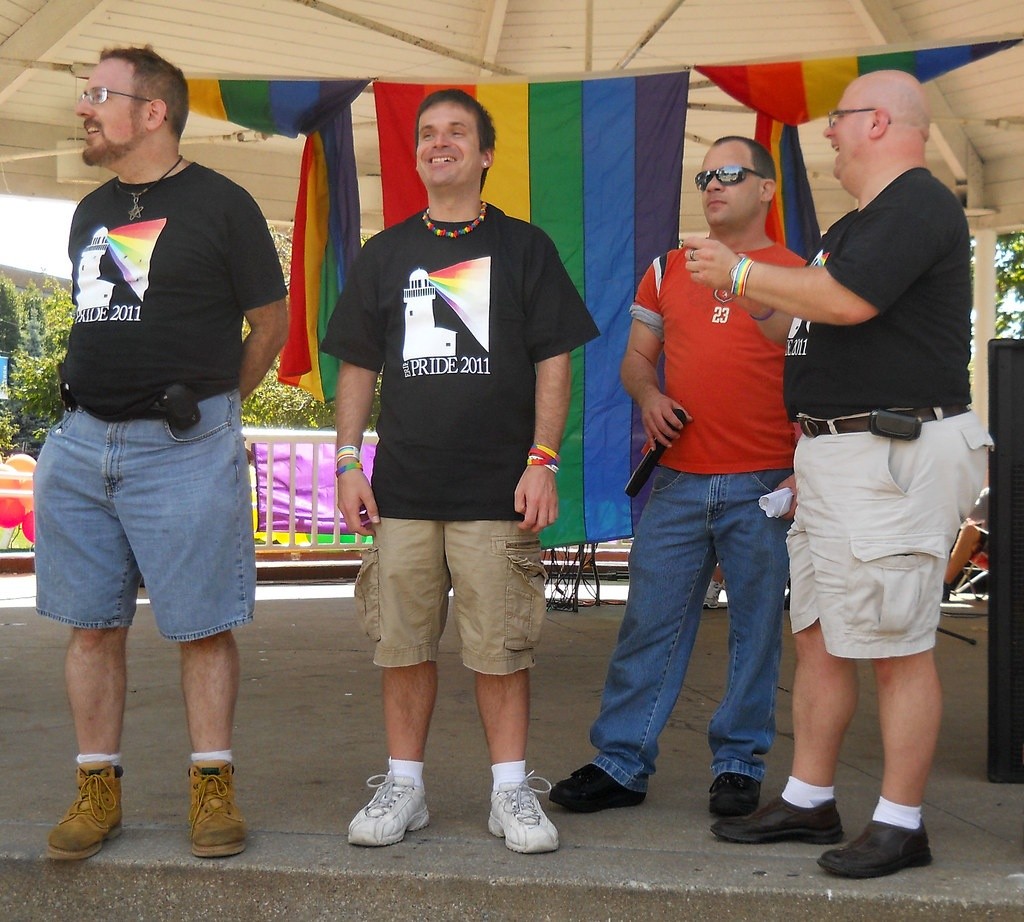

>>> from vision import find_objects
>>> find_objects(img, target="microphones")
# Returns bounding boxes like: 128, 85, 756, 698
623, 409, 687, 497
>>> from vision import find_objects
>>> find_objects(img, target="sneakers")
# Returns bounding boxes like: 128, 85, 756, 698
487, 770, 560, 854
186, 758, 248, 858
347, 755, 430, 848
708, 772, 761, 817
548, 763, 648, 814
46, 760, 124, 861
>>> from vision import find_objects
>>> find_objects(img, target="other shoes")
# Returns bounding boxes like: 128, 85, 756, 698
709, 793, 845, 846
816, 817, 934, 880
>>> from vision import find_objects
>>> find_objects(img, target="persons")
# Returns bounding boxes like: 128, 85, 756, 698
35, 43, 291, 861
684, 70, 996, 879
550, 137, 808, 818
316, 87, 602, 855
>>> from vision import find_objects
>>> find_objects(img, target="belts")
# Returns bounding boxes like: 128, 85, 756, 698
795, 402, 973, 438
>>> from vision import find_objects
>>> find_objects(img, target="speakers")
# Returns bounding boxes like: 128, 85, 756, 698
986, 339, 1024, 784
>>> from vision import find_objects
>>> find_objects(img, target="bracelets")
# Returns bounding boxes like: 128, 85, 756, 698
730, 257, 754, 297
336, 445, 363, 478
731, 266, 738, 282
527, 443, 561, 474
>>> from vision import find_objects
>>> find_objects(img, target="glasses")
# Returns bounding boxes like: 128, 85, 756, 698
827, 107, 891, 128
694, 164, 767, 193
77, 86, 168, 122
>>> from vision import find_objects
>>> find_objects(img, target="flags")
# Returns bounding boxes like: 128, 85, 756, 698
184, 79, 361, 403
371, 64, 690, 548
692, 31, 1024, 262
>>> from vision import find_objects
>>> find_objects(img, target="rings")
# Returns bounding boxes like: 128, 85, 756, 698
690, 249, 696, 261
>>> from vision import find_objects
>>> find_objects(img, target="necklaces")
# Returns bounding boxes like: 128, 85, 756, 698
115, 154, 184, 220
422, 203, 487, 237
